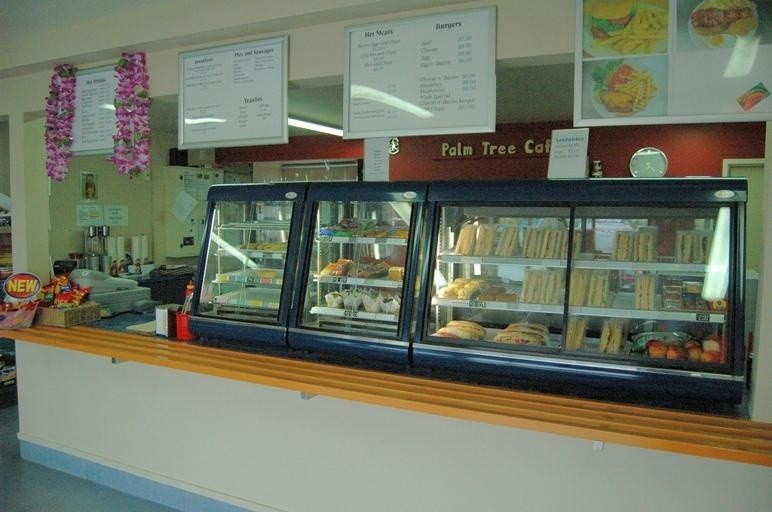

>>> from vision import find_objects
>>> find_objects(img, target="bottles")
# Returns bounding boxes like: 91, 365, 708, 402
112, 259, 126, 276
184, 279, 195, 298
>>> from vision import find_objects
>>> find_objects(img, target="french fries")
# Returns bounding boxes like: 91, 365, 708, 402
610, 10, 667, 54
614, 73, 653, 110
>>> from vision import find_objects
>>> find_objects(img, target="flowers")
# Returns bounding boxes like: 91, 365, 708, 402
40, 60, 79, 183
108, 50, 154, 176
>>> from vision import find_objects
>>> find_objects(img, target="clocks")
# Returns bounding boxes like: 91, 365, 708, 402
628, 145, 671, 179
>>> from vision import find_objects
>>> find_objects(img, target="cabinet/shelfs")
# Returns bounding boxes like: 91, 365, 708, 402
286, 180, 429, 367
149, 164, 226, 269
411, 176, 749, 411
186, 179, 308, 351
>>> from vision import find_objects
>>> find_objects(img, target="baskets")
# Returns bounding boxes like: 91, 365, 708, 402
35, 303, 101, 328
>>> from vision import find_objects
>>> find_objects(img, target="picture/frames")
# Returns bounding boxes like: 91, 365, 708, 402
570, 0, 772, 129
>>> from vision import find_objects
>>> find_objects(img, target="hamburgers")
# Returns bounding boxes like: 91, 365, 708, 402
591, 0, 639, 36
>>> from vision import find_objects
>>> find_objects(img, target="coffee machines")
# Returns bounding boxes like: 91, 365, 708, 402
82, 224, 111, 273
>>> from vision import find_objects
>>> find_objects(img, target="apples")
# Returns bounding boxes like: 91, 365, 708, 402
645, 337, 721, 363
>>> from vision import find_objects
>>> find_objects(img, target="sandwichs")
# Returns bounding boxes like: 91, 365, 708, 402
598, 320, 625, 355
566, 318, 586, 350
454, 225, 713, 309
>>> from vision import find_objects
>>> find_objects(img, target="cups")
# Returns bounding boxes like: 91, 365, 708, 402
129, 264, 140, 273
176, 310, 198, 341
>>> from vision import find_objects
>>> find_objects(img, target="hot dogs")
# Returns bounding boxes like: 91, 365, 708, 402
431, 320, 550, 348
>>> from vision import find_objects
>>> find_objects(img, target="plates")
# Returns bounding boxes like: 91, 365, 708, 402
687, 0, 760, 51
583, 4, 667, 56
591, 61, 656, 119
